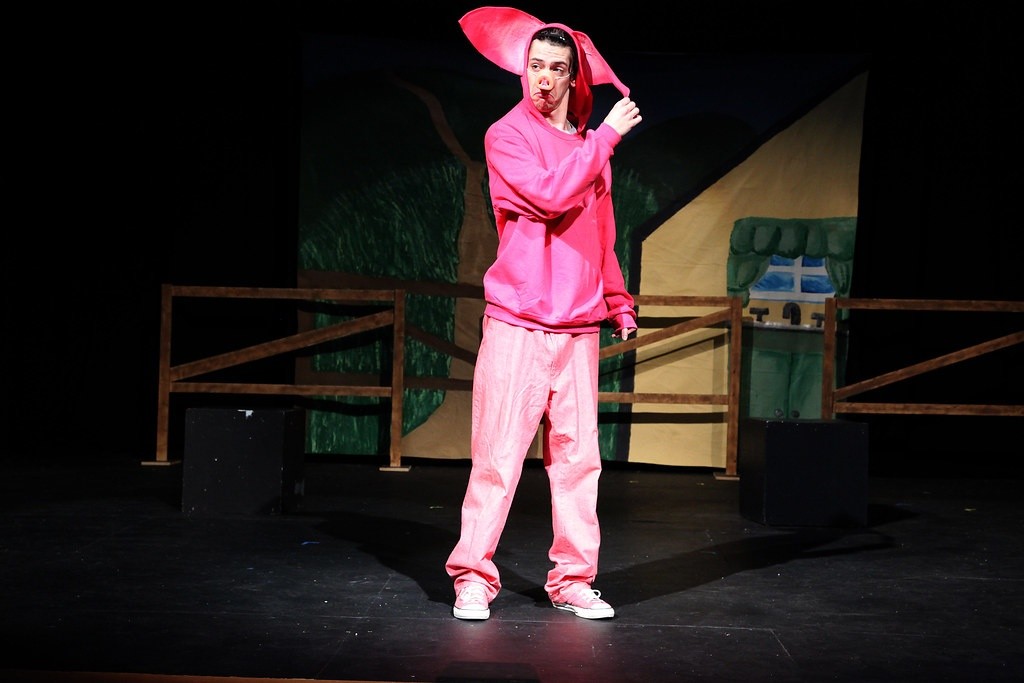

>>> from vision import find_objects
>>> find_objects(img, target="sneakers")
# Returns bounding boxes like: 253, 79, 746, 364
551, 583, 614, 619
453, 582, 490, 619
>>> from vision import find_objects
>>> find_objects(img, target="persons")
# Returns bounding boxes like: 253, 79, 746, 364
443, 22, 645, 620
